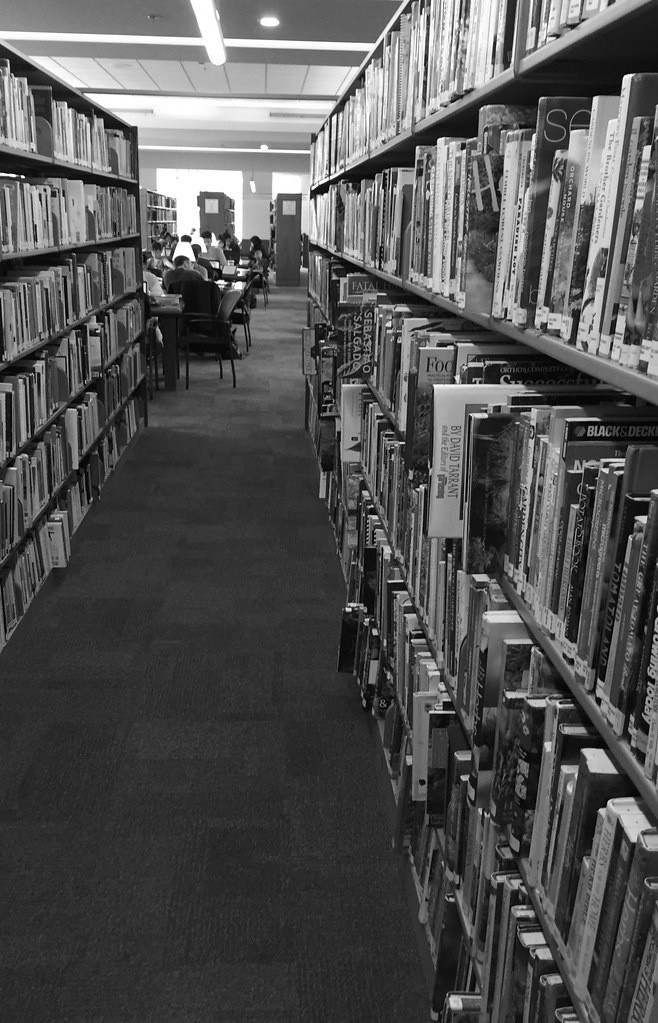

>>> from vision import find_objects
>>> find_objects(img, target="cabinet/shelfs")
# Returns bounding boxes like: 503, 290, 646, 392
0, 40, 150, 655
301, 0, 657, 1023
270, 192, 303, 286
140, 188, 178, 256
197, 191, 235, 246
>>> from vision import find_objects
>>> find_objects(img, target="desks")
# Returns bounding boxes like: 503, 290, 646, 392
150, 288, 185, 392
237, 259, 251, 268
218, 268, 248, 281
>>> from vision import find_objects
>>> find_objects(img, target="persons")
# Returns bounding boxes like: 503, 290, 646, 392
142, 227, 268, 364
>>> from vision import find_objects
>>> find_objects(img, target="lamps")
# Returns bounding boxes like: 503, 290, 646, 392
249, 167, 257, 194
260, 17, 279, 28
190, 1, 227, 66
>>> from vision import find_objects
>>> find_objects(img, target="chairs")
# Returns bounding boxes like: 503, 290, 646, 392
230, 274, 260, 353
166, 279, 222, 361
250, 257, 271, 308
147, 326, 165, 393
177, 290, 243, 390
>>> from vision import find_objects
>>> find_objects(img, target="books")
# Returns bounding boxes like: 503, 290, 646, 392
223, 194, 235, 237
147, 191, 177, 238
302, 1, 658, 1022
0, 59, 147, 654
